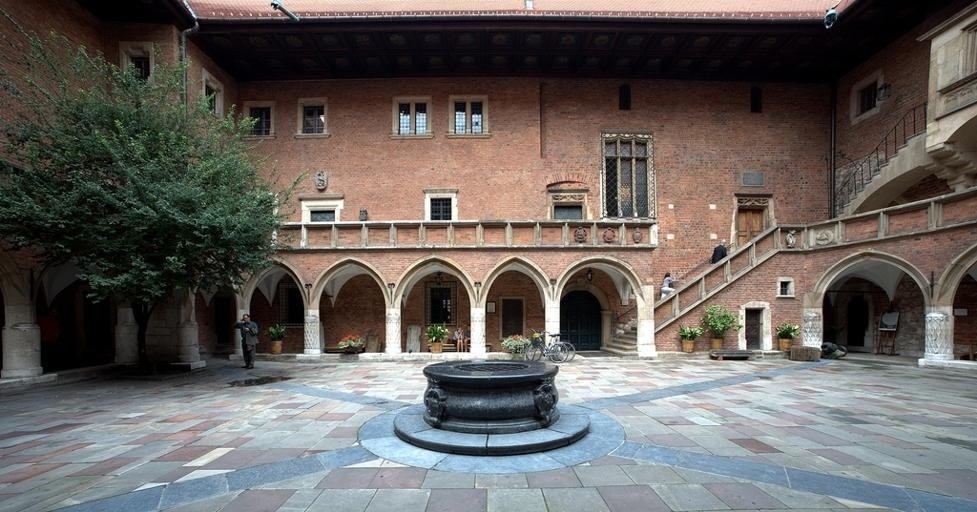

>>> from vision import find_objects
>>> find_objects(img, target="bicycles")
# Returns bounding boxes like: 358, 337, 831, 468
524, 328, 575, 362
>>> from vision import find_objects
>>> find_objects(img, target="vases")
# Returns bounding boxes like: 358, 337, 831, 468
512, 353, 526, 362
778, 338, 792, 352
430, 342, 442, 353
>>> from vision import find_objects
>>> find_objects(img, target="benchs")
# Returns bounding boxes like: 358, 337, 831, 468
424, 332, 492, 352
323, 343, 366, 354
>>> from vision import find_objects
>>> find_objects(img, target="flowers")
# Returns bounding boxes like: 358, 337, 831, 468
500, 334, 532, 354
338, 335, 366, 349
424, 321, 450, 343
773, 321, 802, 340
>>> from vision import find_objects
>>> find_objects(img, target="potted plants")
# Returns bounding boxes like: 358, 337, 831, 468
675, 302, 743, 353
263, 324, 289, 354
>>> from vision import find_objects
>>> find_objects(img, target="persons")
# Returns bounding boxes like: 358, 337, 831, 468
661, 273, 676, 299
231, 314, 260, 369
453, 327, 465, 353
464, 324, 470, 338
712, 239, 727, 264
